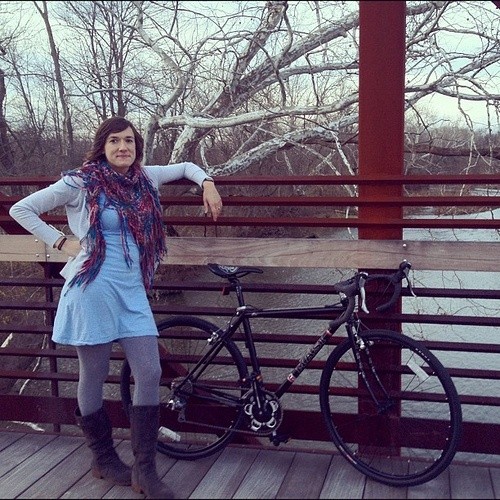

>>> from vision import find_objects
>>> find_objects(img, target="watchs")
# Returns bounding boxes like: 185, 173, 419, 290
202, 177, 214, 189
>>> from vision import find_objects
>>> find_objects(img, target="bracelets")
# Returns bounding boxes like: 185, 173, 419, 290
58, 237, 68, 251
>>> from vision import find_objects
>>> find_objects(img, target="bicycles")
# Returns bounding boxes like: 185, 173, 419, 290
120, 262, 462, 488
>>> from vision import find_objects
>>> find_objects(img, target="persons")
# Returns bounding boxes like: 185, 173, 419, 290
9, 118, 222, 500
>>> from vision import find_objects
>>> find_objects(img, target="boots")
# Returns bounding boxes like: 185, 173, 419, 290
75, 403, 136, 487
129, 402, 174, 500
